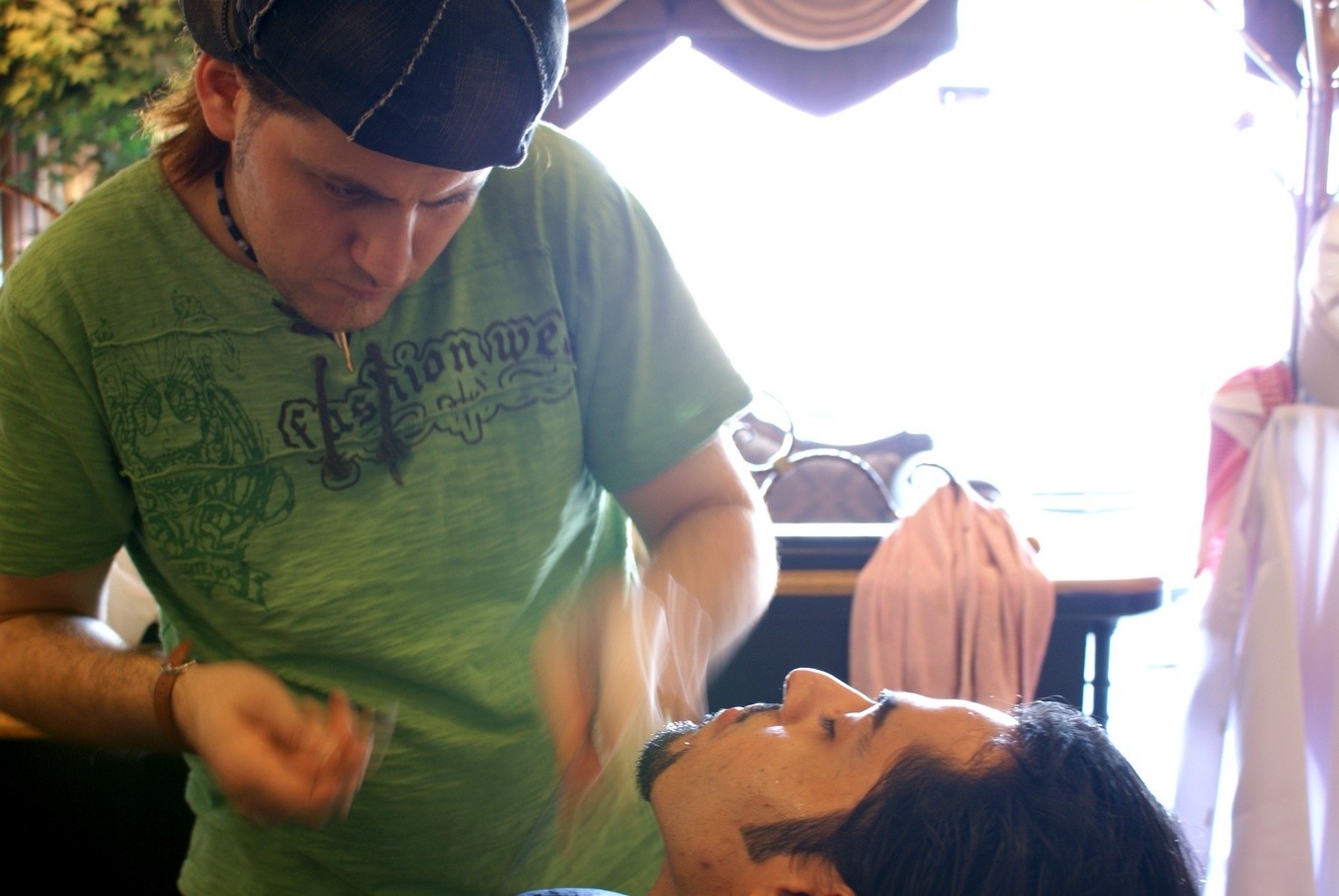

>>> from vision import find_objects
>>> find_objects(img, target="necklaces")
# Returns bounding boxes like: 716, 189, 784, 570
211, 160, 259, 264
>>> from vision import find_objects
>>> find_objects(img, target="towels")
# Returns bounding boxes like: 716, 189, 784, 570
847, 484, 1059, 713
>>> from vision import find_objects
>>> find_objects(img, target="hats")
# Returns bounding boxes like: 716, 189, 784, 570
180, 0, 570, 171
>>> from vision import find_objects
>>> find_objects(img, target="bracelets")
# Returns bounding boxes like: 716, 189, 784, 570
154, 639, 197, 753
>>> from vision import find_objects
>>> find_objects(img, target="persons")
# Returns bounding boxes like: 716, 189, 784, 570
520, 667, 1198, 896
0, 1, 782, 896
1196, 194, 1339, 573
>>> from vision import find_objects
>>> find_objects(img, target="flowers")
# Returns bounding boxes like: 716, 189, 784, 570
0, 0, 199, 191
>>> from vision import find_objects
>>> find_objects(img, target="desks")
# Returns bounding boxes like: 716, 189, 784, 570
766, 567, 1164, 731
